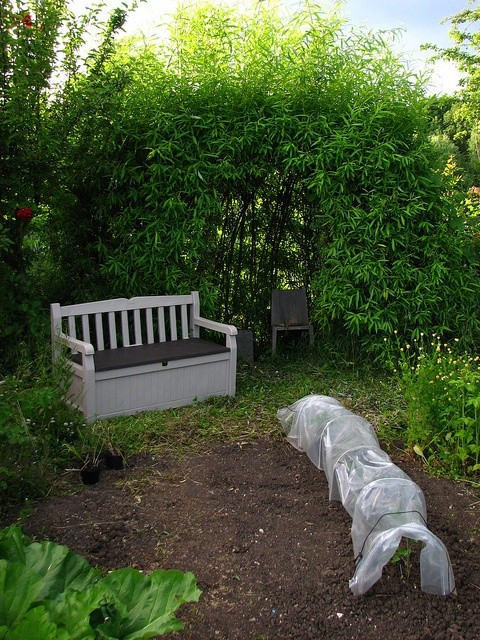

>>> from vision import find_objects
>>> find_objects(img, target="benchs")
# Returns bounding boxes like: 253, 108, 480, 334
50, 291, 238, 427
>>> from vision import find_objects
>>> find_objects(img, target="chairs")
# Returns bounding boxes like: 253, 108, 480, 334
271, 288, 314, 356
